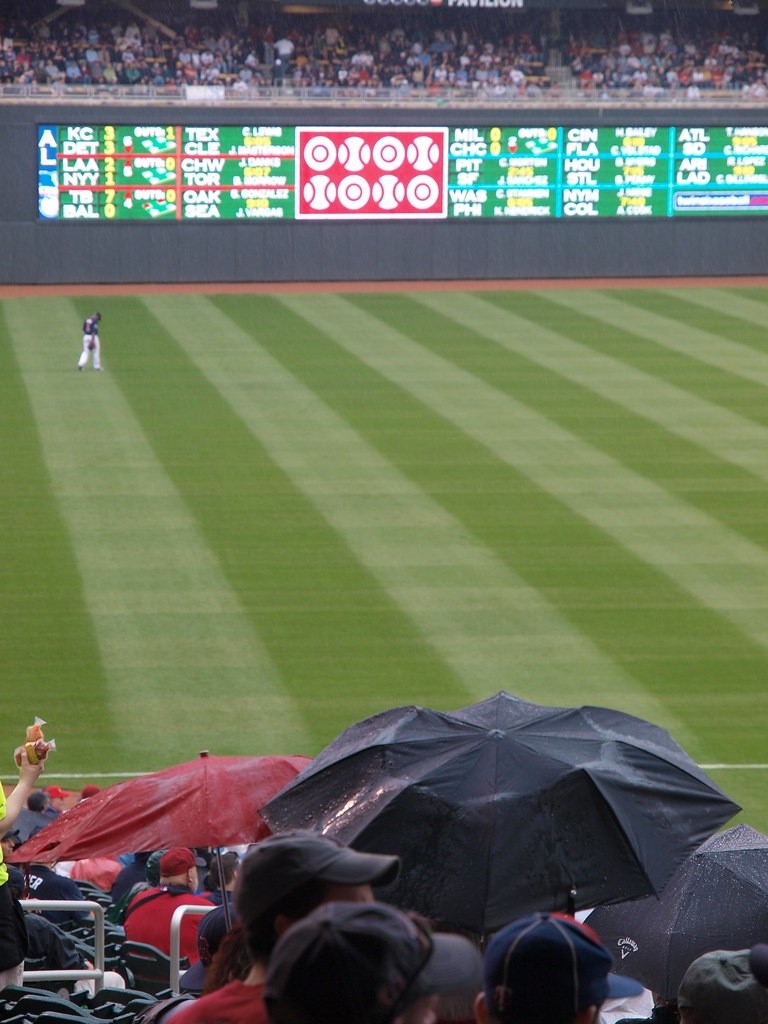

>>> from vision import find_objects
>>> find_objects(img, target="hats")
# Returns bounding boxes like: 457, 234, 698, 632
4, 863, 25, 898
750, 943, 768, 988
45, 785, 70, 801
144, 848, 169, 884
178, 901, 237, 991
1, 828, 20, 840
484, 912, 643, 1019
233, 835, 402, 933
81, 784, 100, 798
159, 847, 207, 877
263, 898, 478, 1024
677, 948, 768, 1024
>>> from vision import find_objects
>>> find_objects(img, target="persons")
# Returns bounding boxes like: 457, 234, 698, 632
0, 828, 99, 953
165, 828, 483, 1024
478, 903, 644, 1024
12, 784, 68, 834
80, 784, 102, 799
122, 849, 217, 964
202, 851, 244, 906
57, 850, 165, 910
679, 950, 768, 1024
77, 311, 105, 372
9, 15, 768, 101
0, 748, 45, 850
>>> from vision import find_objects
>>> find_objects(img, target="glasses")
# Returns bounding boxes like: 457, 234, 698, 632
225, 851, 240, 864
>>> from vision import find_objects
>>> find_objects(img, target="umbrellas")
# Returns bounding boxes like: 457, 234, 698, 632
582, 819, 766, 1007
120, 752, 318, 849
254, 688, 742, 933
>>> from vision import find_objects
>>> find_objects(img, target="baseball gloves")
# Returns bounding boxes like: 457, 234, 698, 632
87, 341, 95, 351
